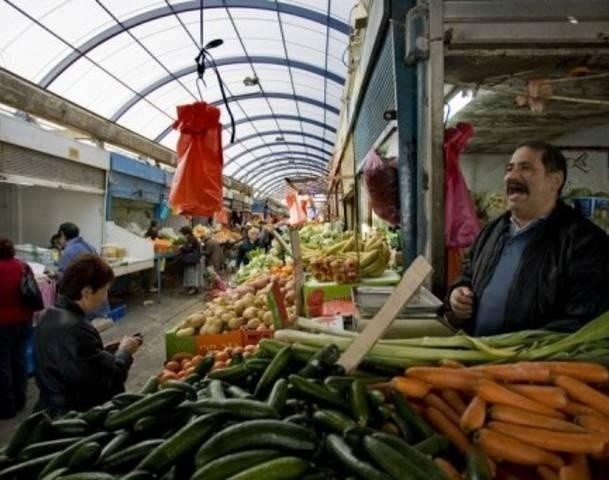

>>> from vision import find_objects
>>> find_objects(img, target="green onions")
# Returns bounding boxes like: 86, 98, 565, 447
273, 310, 609, 365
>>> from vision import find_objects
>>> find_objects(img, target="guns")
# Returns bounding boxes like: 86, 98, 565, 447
326, 233, 391, 278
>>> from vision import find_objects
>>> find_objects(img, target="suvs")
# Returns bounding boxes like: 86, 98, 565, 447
14, 243, 36, 262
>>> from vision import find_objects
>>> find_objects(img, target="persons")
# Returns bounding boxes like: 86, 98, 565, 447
152, 226, 201, 289
48, 233, 67, 252
434, 140, 609, 337
30, 251, 143, 422
0, 235, 35, 422
143, 219, 163, 240
258, 221, 273, 255
235, 227, 268, 270
44, 221, 114, 321
230, 228, 249, 250
230, 211, 242, 227
271, 217, 283, 234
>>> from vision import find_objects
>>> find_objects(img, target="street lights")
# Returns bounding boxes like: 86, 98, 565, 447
19, 273, 44, 311
183, 250, 199, 265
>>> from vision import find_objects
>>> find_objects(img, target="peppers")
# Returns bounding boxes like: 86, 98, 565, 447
175, 265, 297, 333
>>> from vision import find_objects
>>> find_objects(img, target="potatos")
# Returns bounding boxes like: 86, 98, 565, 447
175, 265, 297, 333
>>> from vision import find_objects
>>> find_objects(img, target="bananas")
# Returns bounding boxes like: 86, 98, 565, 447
326, 233, 391, 278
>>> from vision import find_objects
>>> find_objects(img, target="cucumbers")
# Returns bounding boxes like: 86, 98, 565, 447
0, 339, 489, 480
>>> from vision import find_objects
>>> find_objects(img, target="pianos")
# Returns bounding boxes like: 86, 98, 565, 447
389, 357, 609, 480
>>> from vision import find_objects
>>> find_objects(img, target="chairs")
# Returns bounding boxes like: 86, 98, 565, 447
148, 288, 157, 292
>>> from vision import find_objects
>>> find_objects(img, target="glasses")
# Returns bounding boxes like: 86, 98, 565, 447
244, 75, 257, 86
348, 0, 368, 28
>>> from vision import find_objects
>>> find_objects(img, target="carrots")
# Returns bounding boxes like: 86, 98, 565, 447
389, 357, 609, 480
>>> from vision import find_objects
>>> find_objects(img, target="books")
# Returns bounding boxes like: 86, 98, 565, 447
167, 325, 275, 362
